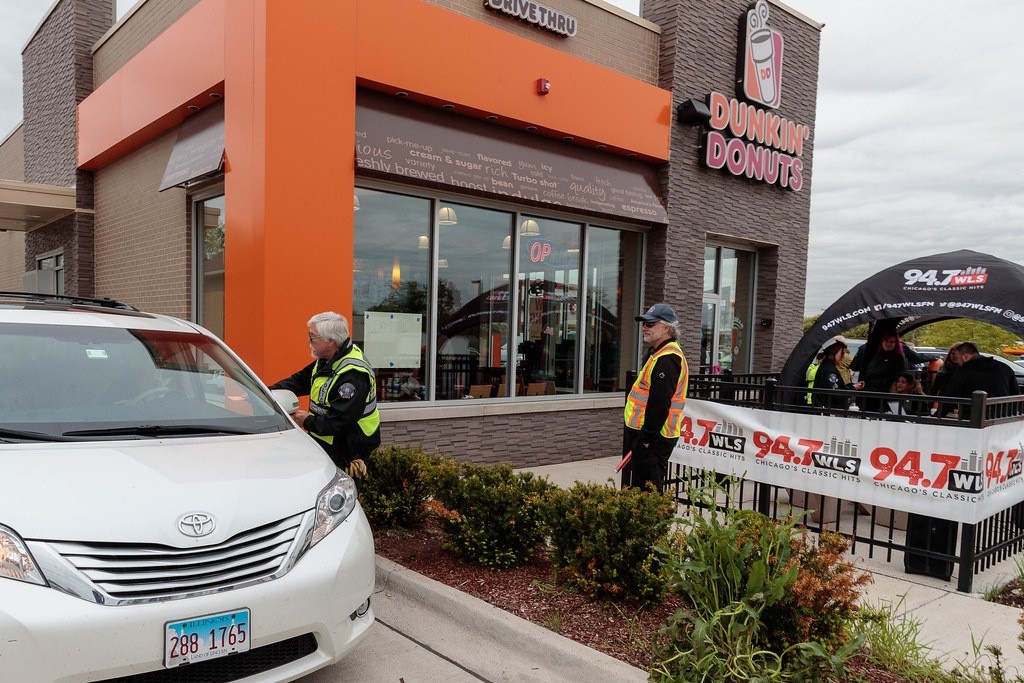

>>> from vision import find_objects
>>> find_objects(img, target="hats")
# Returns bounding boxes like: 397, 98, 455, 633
635, 303, 677, 323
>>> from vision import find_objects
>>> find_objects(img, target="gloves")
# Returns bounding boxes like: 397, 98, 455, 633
345, 459, 369, 481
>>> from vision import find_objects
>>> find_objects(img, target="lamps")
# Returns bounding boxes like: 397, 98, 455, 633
419, 234, 428, 250
440, 207, 458, 226
501, 235, 512, 249
439, 255, 450, 269
677, 98, 713, 126
520, 220, 541, 236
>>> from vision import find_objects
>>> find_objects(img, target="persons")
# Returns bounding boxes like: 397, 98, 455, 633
267, 311, 382, 489
804, 318, 1022, 420
623, 304, 690, 497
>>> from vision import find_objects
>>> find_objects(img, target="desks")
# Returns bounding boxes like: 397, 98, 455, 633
524, 387, 602, 393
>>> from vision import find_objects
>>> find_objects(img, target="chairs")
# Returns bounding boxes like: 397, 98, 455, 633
469, 384, 493, 398
585, 378, 593, 389
497, 374, 525, 396
526, 383, 547, 395
536, 380, 556, 394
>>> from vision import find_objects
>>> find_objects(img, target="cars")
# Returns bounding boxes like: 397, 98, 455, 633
839, 339, 1024, 408
0, 290, 384, 683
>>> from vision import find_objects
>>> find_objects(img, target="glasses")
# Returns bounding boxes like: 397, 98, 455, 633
308, 330, 321, 343
643, 321, 660, 328
